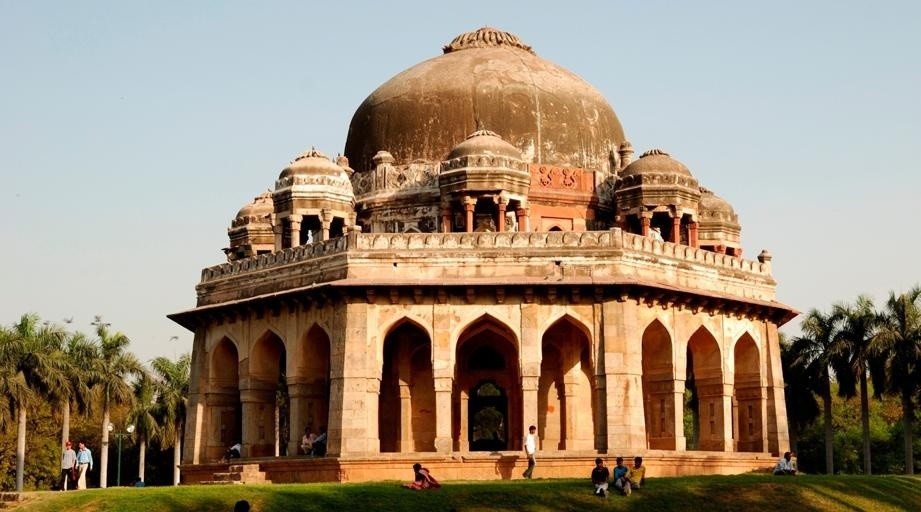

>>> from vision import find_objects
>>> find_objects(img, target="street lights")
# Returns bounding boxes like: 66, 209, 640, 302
108, 422, 136, 487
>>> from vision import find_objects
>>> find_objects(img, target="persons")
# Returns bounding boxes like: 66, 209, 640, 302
134, 477, 146, 487
74, 439, 94, 489
310, 425, 327, 457
841, 456, 854, 474
591, 457, 610, 499
403, 463, 441, 490
233, 500, 250, 512
301, 427, 317, 454
523, 425, 537, 479
773, 451, 797, 475
622, 456, 646, 489
614, 457, 631, 498
226, 439, 241, 458
59, 441, 76, 492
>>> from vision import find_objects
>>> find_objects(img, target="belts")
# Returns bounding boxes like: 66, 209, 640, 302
78, 462, 88, 464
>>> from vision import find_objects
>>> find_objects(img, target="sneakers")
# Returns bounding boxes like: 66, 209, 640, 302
522, 473, 532, 479
595, 487, 632, 498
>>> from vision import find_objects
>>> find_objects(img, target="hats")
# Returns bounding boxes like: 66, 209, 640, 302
65, 442, 72, 447
785, 451, 793, 456
